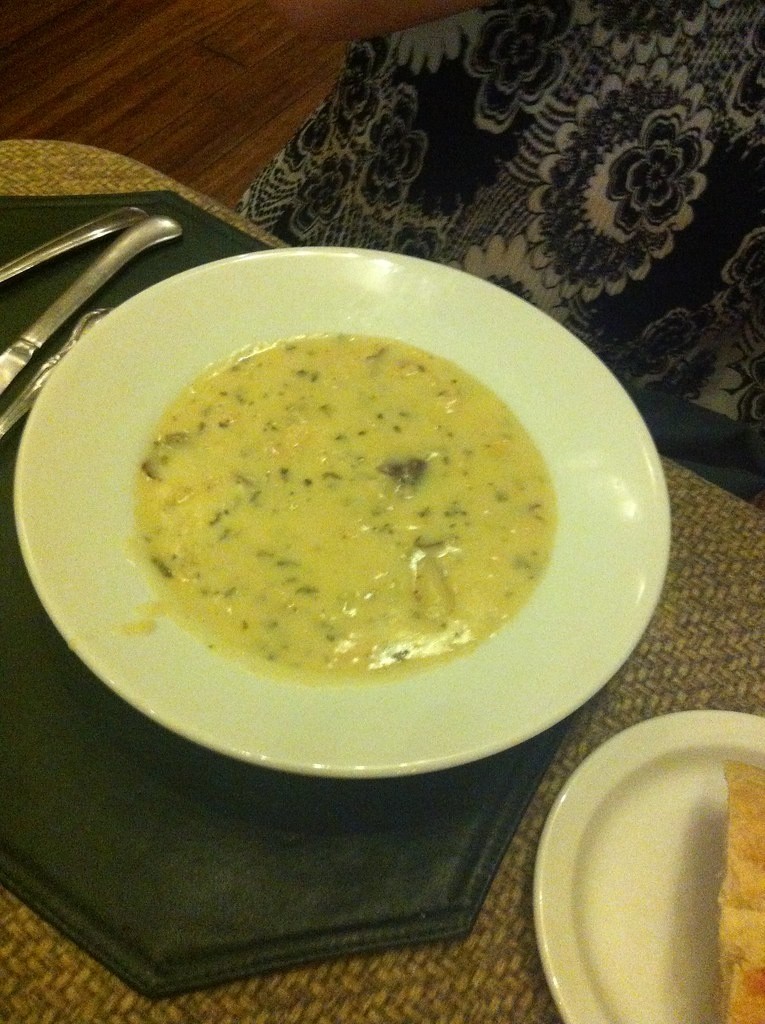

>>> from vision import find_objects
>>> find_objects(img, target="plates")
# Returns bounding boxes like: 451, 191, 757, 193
12, 246, 671, 776
530, 708, 764, 1024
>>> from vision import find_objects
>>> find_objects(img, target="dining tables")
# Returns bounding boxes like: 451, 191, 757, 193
0, 136, 765, 1024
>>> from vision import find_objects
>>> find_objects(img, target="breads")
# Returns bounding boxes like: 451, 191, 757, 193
711, 759, 765, 1024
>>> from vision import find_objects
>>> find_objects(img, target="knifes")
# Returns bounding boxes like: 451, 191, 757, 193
0, 217, 181, 406
